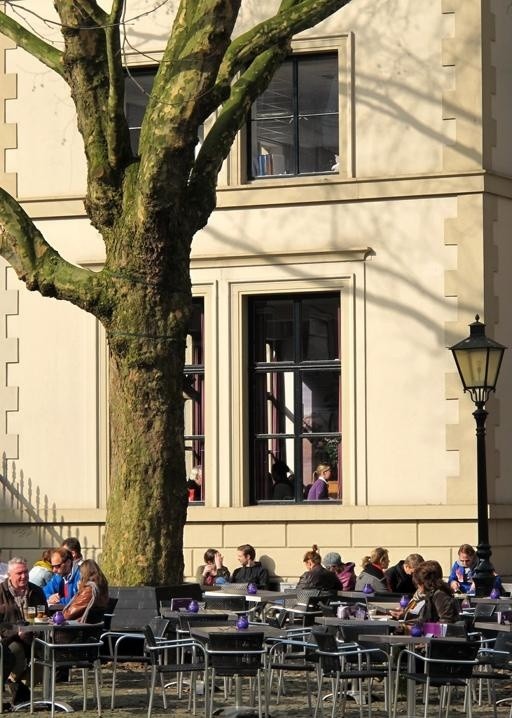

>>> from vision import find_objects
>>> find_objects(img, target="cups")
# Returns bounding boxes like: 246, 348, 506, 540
398, 620, 406, 634
356, 603, 377, 619
423, 623, 440, 636
37, 605, 45, 617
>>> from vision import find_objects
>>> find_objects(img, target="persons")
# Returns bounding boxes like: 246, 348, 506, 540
311, 437, 331, 471
196, 548, 230, 586
271, 462, 294, 500
296, 544, 506, 695
0, 538, 109, 713
186, 464, 204, 501
306, 463, 332, 500
232, 544, 268, 586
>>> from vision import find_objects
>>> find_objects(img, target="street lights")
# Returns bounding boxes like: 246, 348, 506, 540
449, 314, 509, 591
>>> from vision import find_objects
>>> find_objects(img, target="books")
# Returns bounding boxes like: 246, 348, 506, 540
255, 154, 287, 176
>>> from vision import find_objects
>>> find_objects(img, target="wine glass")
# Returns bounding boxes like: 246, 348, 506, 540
28, 607, 35, 626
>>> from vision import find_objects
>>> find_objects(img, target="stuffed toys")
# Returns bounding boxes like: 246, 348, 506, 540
331, 153, 340, 172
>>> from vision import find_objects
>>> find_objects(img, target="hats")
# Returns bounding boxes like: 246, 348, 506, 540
322, 552, 342, 566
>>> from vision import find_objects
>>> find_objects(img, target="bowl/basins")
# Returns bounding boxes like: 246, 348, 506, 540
34, 617, 48, 622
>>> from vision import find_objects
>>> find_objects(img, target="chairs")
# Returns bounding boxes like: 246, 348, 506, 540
0, 579, 512, 718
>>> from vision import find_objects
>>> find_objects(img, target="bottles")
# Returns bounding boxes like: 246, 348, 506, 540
400, 593, 409, 607
248, 584, 257, 594
189, 600, 198, 613
490, 587, 500, 599
439, 619, 447, 637
237, 615, 249, 631
53, 611, 63, 624
411, 623, 421, 637
364, 584, 372, 593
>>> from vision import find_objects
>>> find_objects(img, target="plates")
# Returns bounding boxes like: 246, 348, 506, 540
34, 622, 49, 624
499, 596, 511, 600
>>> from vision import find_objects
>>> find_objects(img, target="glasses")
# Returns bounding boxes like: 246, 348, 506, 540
49, 559, 68, 569
